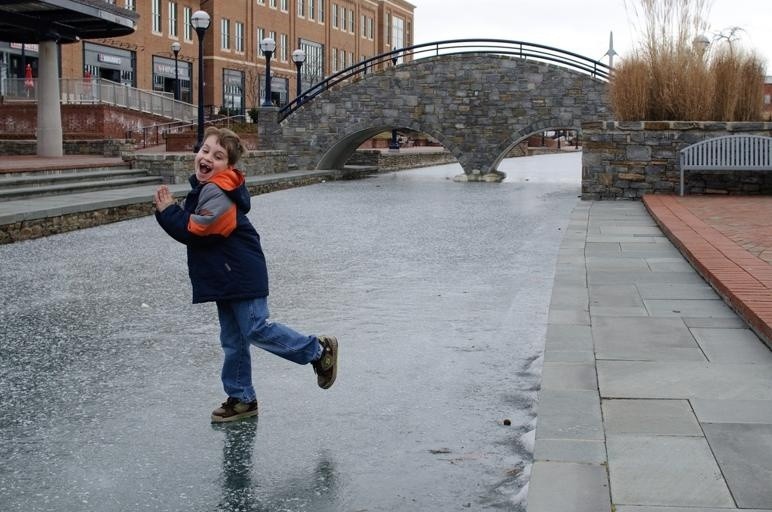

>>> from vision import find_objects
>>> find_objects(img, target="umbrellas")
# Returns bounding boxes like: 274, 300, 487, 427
24, 63, 34, 99
83, 71, 93, 93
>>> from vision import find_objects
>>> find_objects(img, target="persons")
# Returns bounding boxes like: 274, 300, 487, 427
391, 46, 400, 66
152, 127, 338, 423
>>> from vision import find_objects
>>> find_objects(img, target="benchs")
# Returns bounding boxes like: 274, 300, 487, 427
680, 135, 772, 196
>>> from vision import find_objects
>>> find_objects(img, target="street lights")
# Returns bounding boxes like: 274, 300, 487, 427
389, 47, 399, 150
171, 42, 181, 102
189, 9, 212, 153
691, 33, 710, 62
259, 38, 278, 107
291, 49, 306, 105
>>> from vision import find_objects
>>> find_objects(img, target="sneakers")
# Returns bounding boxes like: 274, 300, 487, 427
311, 335, 338, 390
211, 397, 258, 422
